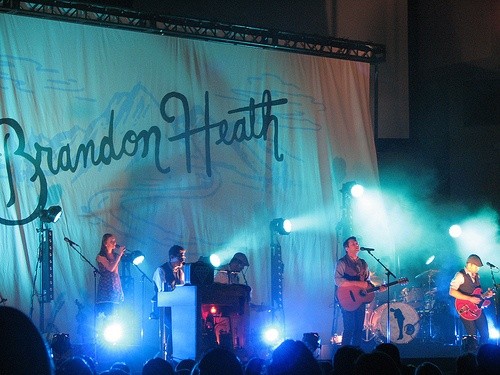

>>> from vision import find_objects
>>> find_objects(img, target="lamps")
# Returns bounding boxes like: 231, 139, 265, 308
201, 254, 220, 267
272, 218, 292, 236
343, 182, 363, 198
129, 251, 145, 264
43, 205, 62, 223
420, 251, 435, 264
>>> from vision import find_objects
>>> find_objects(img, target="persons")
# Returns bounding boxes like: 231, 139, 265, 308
152, 244, 186, 356
334, 236, 388, 346
214, 252, 250, 284
96, 233, 125, 342
449, 254, 493, 354
0, 306, 500, 375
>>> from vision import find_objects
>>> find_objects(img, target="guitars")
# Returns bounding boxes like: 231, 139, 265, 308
335, 276, 410, 313
455, 287, 497, 321
251, 302, 270, 313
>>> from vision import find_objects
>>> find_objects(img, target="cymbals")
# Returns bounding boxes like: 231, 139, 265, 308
415, 269, 440, 279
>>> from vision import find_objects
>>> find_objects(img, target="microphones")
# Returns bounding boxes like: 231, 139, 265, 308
360, 247, 374, 251
407, 327, 412, 331
64, 237, 79, 246
115, 245, 129, 251
486, 262, 498, 269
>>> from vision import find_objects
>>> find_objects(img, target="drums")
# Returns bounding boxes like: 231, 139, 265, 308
364, 308, 375, 331
401, 285, 425, 307
370, 278, 387, 287
370, 300, 421, 344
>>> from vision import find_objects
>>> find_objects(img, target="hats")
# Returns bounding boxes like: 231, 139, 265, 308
468, 255, 483, 267
